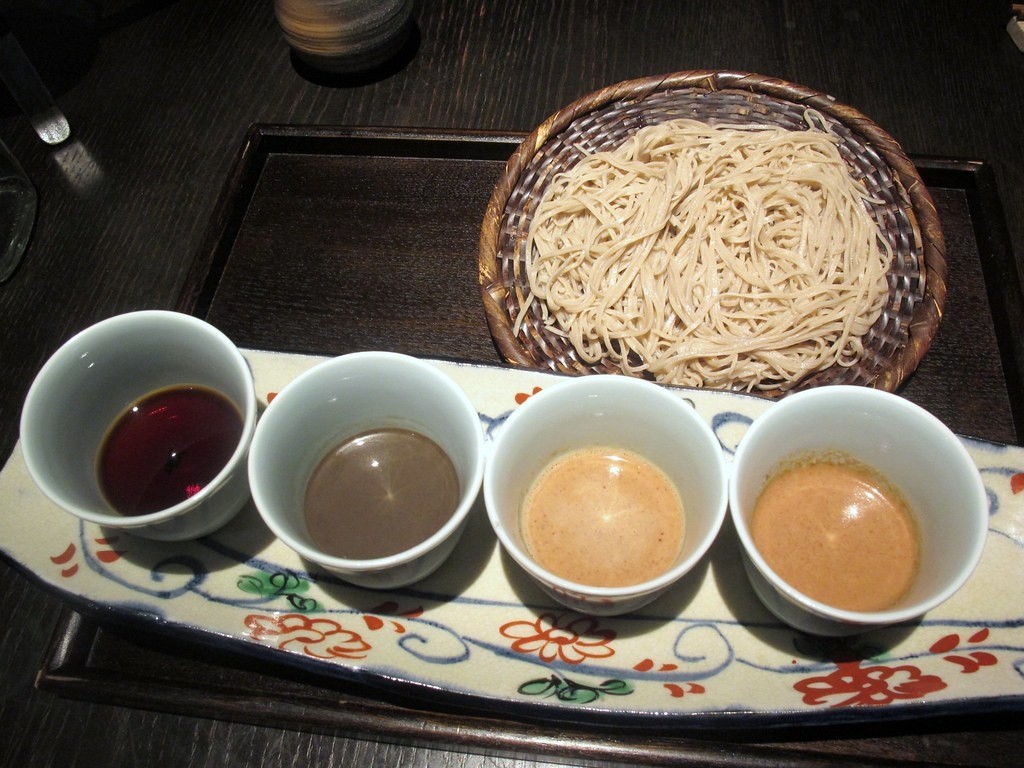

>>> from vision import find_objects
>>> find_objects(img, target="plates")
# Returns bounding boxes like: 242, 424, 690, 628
0, 348, 1024, 717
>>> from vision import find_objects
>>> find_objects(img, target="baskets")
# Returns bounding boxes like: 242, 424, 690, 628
478, 68, 945, 402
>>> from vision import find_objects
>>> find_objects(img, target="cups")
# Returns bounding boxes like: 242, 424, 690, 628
19, 309, 258, 543
483, 374, 729, 617
247, 351, 486, 589
728, 385, 989, 638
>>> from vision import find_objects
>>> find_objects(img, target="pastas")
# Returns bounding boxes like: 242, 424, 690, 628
513, 108, 893, 393
